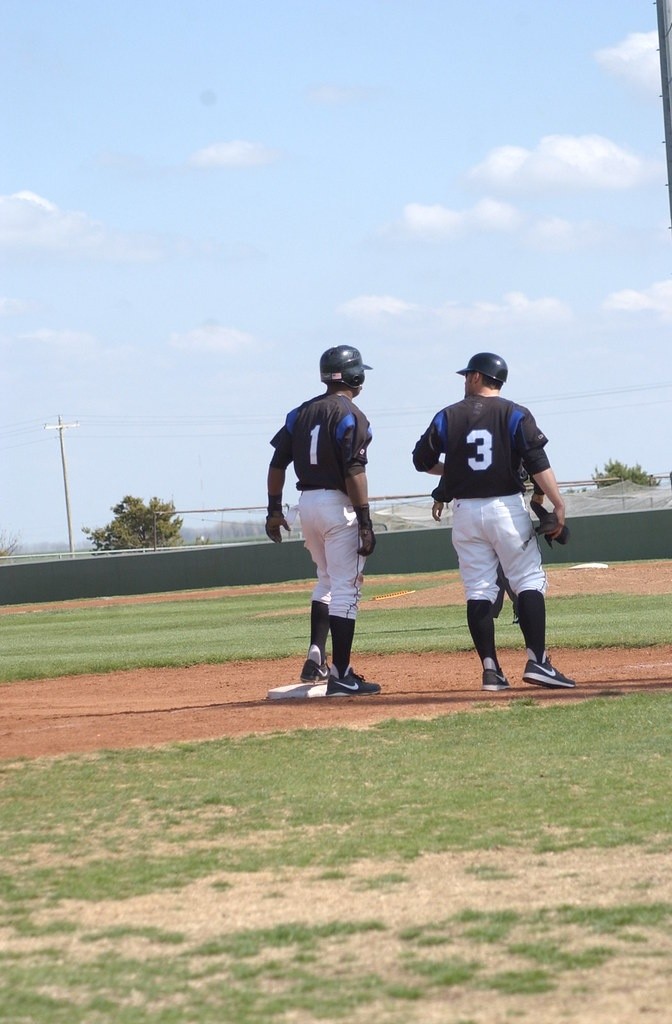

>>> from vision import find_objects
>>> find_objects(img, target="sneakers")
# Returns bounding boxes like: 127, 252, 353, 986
302, 659, 330, 684
481, 668, 510, 691
523, 655, 576, 688
327, 668, 381, 697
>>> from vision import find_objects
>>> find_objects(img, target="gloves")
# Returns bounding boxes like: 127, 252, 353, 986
265, 493, 291, 543
353, 504, 376, 557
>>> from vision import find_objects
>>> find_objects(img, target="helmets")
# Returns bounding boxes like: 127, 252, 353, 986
456, 352, 507, 383
319, 344, 373, 389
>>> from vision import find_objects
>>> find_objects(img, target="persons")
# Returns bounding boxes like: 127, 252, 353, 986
263, 347, 386, 697
431, 469, 552, 624
411, 351, 578, 694
